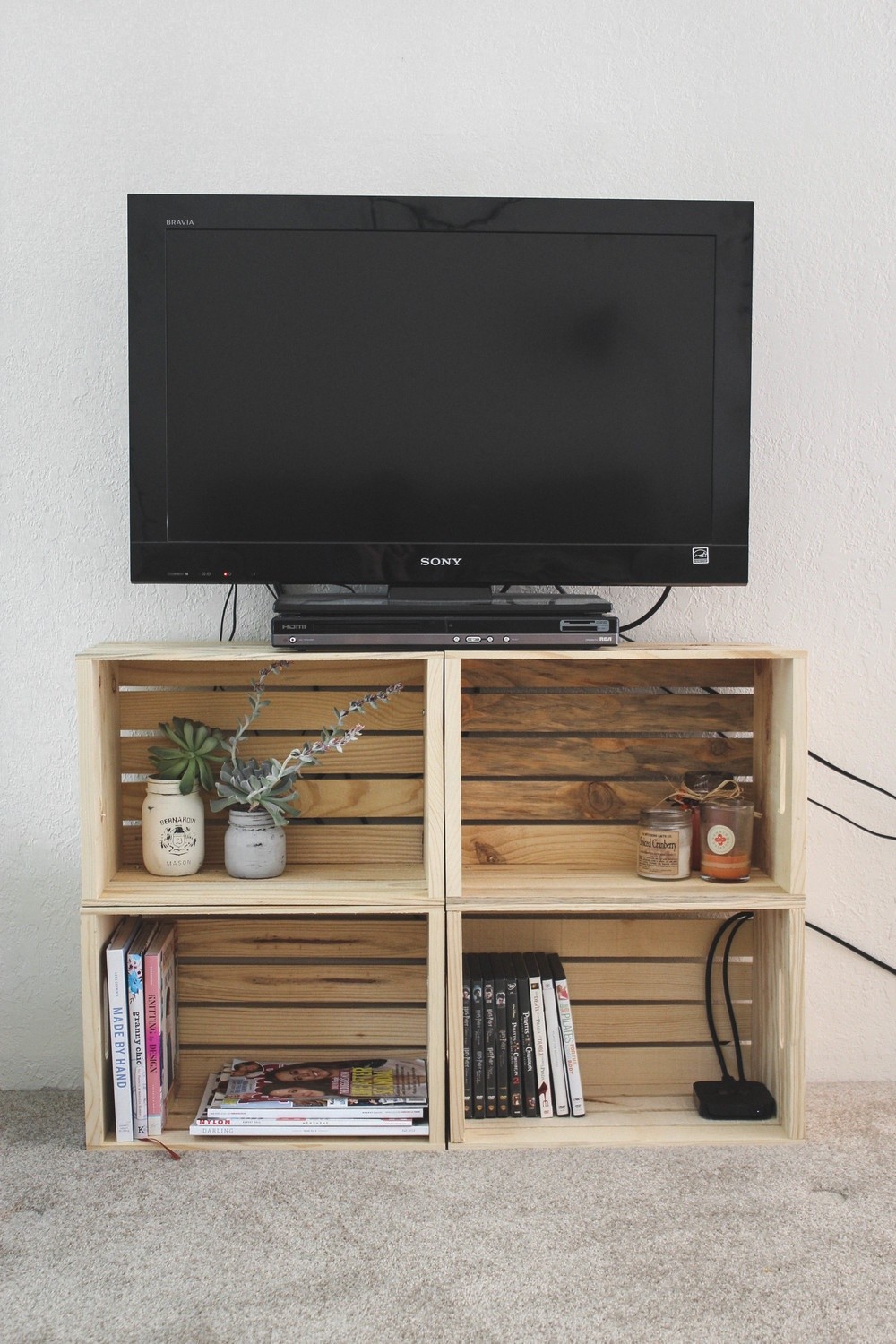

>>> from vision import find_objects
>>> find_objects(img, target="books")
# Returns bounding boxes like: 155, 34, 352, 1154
190, 1063, 430, 1137
220, 1057, 429, 1109
106, 916, 178, 1144
463, 952, 586, 1119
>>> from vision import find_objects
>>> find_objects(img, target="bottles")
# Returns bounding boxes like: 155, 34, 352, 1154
637, 808, 691, 879
678, 772, 734, 870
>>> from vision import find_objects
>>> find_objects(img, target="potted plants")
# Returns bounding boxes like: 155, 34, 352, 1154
144, 712, 229, 875
209, 662, 405, 877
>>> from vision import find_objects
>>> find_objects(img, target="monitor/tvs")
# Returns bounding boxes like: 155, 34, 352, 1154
127, 193, 752, 615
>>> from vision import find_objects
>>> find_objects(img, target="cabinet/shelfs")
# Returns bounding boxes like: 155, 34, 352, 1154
76, 643, 806, 1151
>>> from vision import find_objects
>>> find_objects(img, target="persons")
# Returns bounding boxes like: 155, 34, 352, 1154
463, 989, 470, 1004
473, 989, 482, 1001
557, 984, 569, 1000
485, 989, 492, 1000
497, 994, 505, 1007
230, 1059, 387, 1105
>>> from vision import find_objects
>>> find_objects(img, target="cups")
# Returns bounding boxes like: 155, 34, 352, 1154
699, 799, 755, 884
